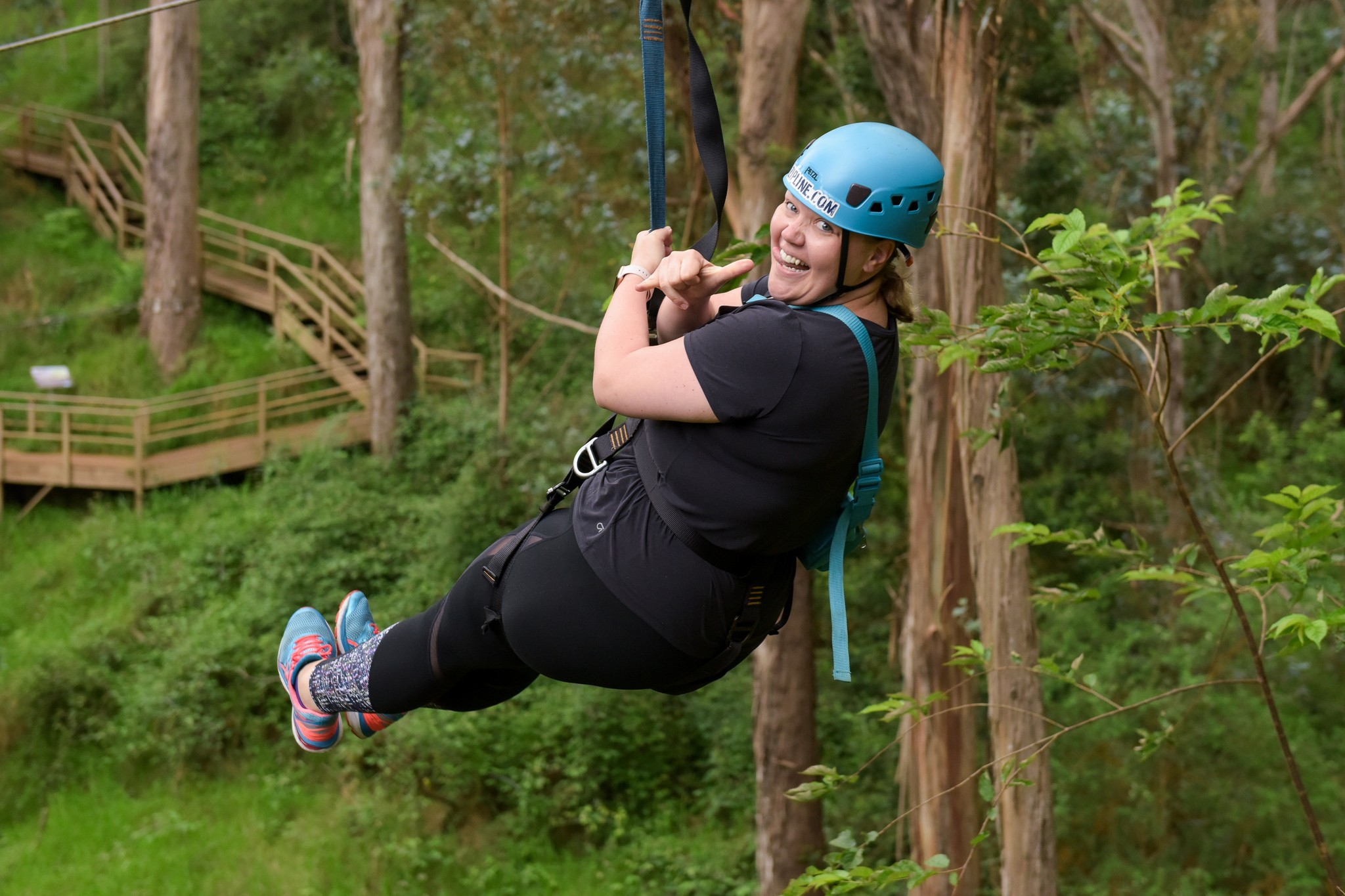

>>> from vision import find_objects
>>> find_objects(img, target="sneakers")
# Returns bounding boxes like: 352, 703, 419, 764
276, 606, 344, 756
334, 588, 406, 739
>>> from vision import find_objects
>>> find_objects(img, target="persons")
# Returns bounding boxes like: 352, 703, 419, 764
275, 124, 942, 755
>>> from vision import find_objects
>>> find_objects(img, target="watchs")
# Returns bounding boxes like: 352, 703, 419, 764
613, 263, 655, 302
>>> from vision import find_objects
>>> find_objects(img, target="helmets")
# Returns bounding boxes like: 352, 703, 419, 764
782, 119, 945, 250
